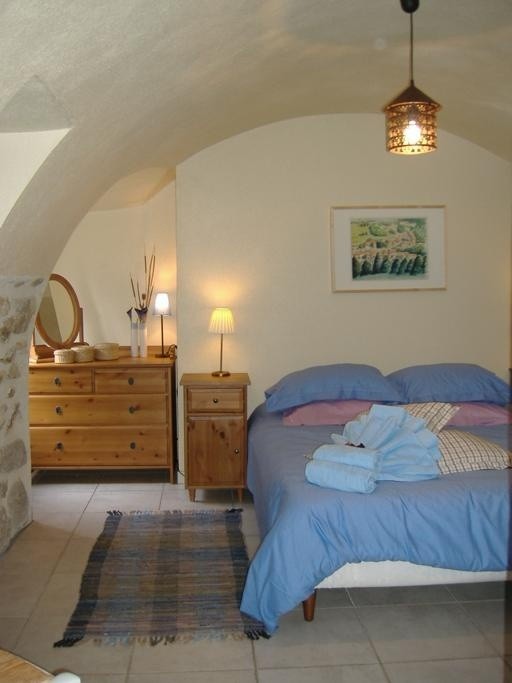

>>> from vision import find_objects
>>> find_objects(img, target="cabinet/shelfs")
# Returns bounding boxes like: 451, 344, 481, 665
28, 344, 176, 485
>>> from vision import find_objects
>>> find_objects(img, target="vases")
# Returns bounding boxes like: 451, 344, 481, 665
135, 307, 149, 358
127, 307, 139, 357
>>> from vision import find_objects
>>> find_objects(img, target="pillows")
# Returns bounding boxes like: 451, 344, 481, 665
392, 402, 462, 439
428, 431, 512, 473
265, 363, 400, 412
281, 398, 378, 426
437, 403, 510, 428
385, 362, 510, 403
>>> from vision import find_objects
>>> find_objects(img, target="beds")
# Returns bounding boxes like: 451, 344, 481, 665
253, 392, 511, 621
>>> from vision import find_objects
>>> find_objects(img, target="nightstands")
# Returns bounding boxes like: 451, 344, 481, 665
179, 373, 251, 503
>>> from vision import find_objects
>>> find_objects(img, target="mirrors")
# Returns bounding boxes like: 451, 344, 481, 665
30, 273, 89, 363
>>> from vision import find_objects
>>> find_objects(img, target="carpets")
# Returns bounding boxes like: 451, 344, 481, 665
53, 508, 277, 649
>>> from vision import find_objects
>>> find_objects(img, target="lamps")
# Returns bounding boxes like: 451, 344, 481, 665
208, 308, 236, 377
382, 1, 441, 156
153, 292, 172, 358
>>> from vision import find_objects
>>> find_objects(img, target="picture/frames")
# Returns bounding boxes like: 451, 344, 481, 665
329, 203, 448, 292
